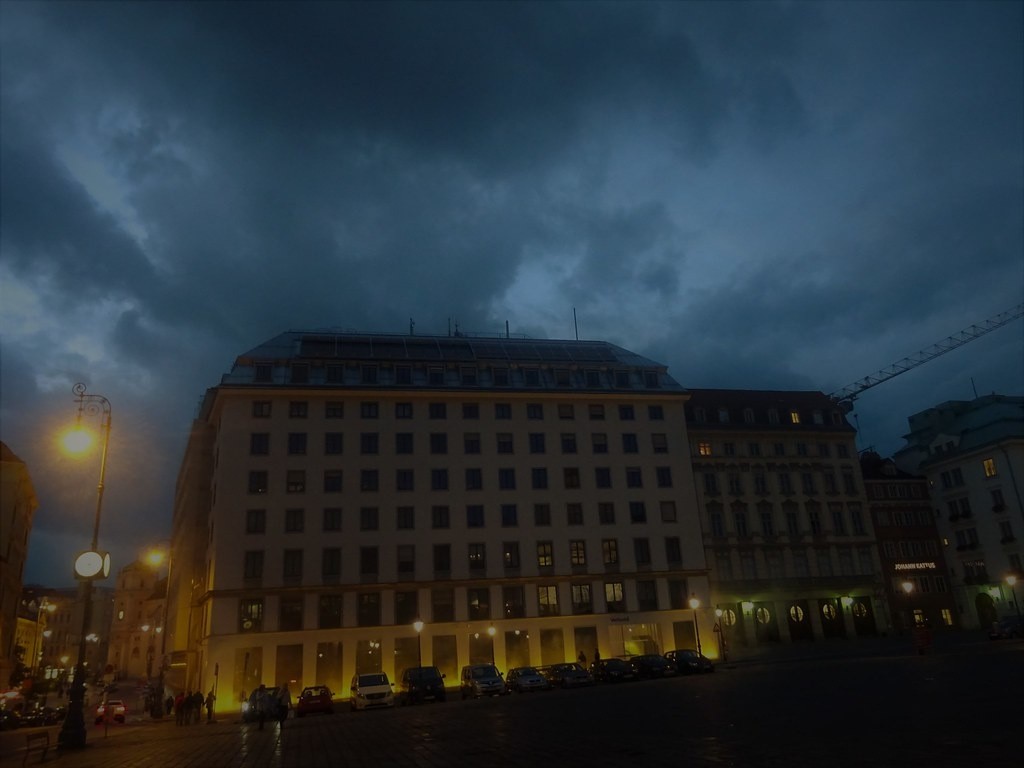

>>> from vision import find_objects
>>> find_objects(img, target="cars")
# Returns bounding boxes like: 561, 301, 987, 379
242, 685, 288, 726
664, 649, 714, 675
296, 685, 336, 717
545, 663, 597, 689
0, 705, 66, 731
94, 699, 126, 725
988, 621, 1021, 640
505, 666, 552, 694
590, 658, 639, 684
630, 655, 678, 681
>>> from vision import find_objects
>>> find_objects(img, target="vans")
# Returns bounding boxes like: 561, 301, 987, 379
401, 666, 447, 707
460, 662, 509, 698
349, 670, 396, 711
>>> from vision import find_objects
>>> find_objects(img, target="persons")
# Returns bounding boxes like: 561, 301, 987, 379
256, 684, 268, 730
578, 651, 586, 663
595, 648, 599, 660
165, 689, 216, 727
276, 683, 292, 730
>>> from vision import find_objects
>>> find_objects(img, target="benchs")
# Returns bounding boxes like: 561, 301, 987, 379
22, 731, 65, 766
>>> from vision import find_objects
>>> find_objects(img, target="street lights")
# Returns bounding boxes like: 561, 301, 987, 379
413, 614, 424, 666
1007, 576, 1020, 616
687, 592, 702, 658
486, 621, 497, 665
54, 382, 113, 753
713, 605, 728, 664
